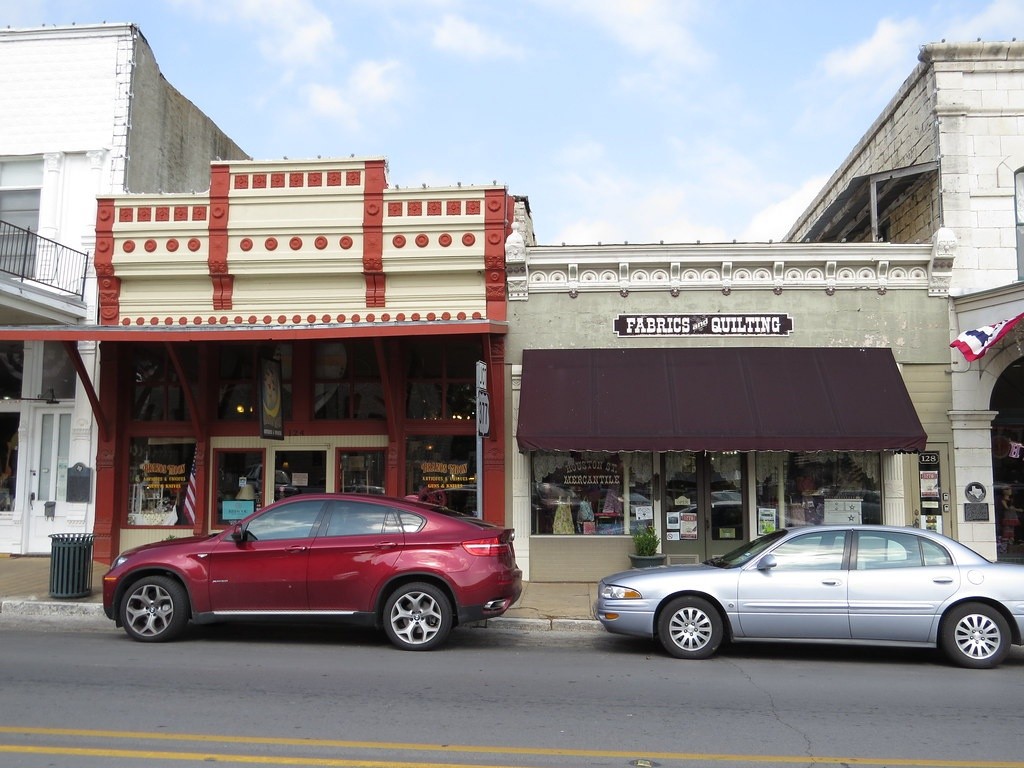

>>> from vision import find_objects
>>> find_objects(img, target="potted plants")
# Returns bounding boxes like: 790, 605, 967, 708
628, 527, 667, 568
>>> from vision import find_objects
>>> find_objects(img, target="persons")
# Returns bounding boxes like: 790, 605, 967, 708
1001, 484, 1024, 546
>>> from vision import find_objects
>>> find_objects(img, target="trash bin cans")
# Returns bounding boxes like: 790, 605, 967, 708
48, 532, 98, 599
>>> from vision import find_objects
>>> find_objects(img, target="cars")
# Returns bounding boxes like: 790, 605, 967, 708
595, 524, 1023, 671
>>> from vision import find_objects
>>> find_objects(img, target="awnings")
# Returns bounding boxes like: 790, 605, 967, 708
518, 347, 929, 454
0, 322, 511, 341
799, 160, 939, 243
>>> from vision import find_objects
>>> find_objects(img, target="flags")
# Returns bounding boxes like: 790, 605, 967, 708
949, 311, 1024, 361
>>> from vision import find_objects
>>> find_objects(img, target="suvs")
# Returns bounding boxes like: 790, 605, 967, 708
101, 489, 520, 650
